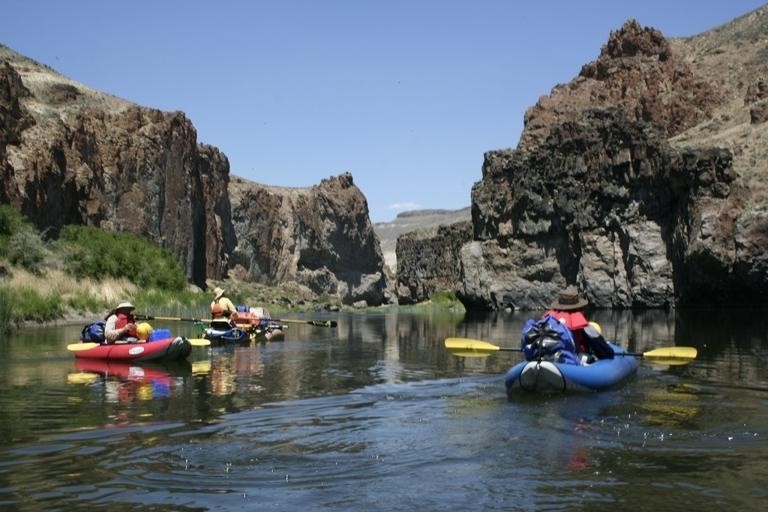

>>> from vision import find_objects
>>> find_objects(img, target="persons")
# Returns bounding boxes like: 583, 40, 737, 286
210, 287, 239, 330
538, 288, 615, 364
103, 302, 137, 345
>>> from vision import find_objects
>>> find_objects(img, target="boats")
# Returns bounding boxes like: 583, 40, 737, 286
503, 340, 638, 393
74, 305, 285, 361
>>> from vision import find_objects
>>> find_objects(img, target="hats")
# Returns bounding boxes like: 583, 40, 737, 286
213, 286, 227, 301
116, 302, 136, 311
550, 288, 589, 310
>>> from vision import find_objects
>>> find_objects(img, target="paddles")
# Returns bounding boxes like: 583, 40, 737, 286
66, 337, 210, 351
444, 337, 698, 365
129, 312, 338, 329
